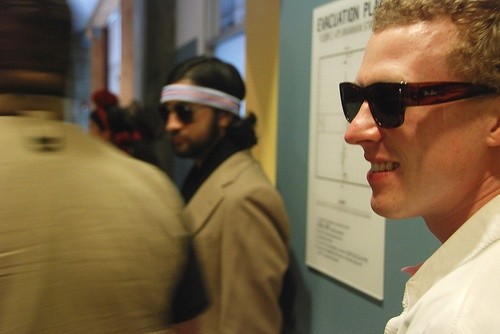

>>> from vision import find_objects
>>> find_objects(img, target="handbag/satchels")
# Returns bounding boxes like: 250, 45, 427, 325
245, 194, 310, 334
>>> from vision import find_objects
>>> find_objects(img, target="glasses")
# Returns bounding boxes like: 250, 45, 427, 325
338, 79, 497, 129
158, 102, 209, 126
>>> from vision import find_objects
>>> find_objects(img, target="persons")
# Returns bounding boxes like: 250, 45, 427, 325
160, 56, 294, 334
343, 0, 500, 334
0, 1, 216, 334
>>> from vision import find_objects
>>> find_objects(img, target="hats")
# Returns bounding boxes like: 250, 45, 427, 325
0, 0, 76, 97
